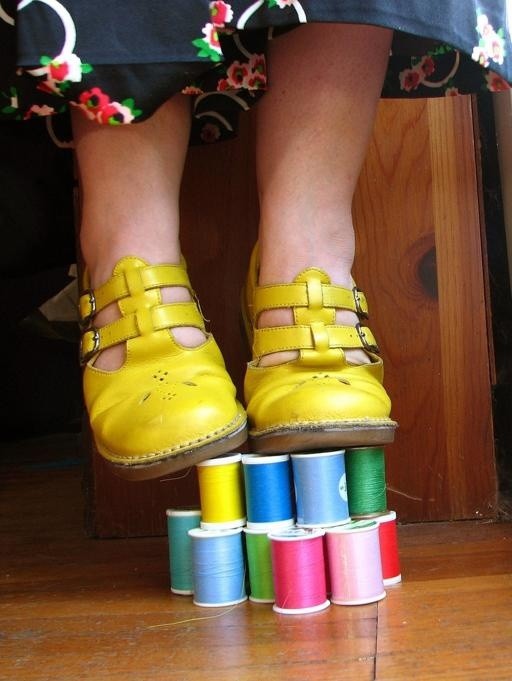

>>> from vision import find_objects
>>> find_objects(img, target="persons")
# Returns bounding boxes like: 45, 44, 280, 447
1, 1, 512, 484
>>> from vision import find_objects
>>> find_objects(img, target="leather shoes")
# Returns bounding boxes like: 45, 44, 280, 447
241, 237, 397, 454
80, 254, 248, 481
